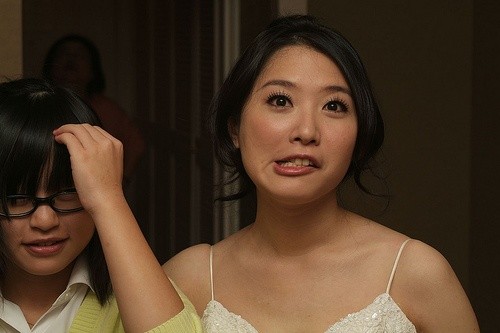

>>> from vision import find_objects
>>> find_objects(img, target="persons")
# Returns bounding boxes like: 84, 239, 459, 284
0, 78, 202, 333
41, 34, 143, 171
160, 16, 482, 333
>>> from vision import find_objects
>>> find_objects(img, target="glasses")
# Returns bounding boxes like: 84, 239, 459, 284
0, 188, 85, 218
